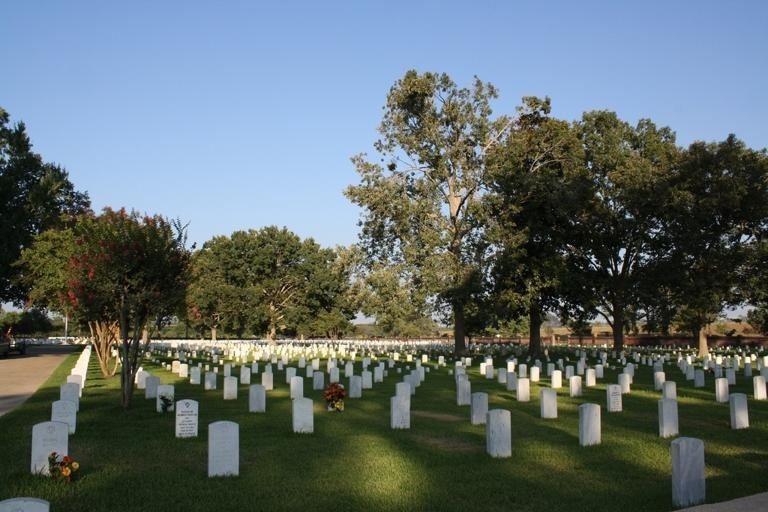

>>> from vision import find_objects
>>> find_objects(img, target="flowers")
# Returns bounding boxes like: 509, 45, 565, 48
323, 378, 347, 415
159, 392, 175, 414
46, 451, 82, 482
